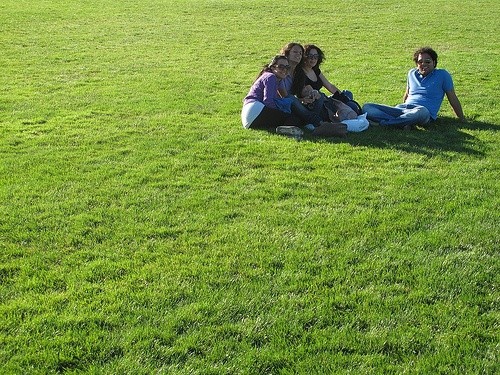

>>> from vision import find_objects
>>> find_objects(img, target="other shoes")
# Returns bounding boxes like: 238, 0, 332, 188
399, 124, 416, 131
276, 125, 304, 137
341, 120, 369, 132
368, 118, 373, 127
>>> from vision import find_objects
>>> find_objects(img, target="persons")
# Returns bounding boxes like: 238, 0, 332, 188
362, 46, 474, 130
240, 42, 352, 128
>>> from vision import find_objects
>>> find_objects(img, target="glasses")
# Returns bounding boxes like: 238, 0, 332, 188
307, 54, 319, 59
274, 64, 291, 69
